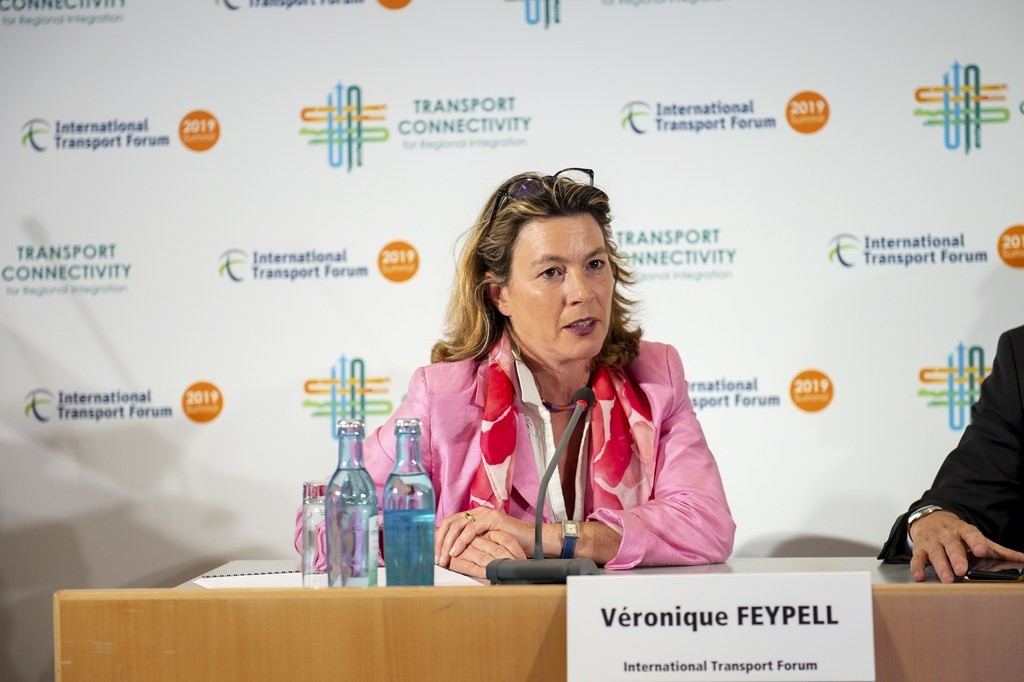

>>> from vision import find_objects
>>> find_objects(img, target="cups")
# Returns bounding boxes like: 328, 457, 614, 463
302, 483, 328, 589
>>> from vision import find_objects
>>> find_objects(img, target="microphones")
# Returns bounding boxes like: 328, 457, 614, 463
486, 386, 601, 584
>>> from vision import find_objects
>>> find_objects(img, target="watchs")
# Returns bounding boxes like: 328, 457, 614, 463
906, 504, 956, 542
558, 519, 580, 559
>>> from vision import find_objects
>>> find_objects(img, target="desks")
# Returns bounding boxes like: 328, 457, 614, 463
53, 557, 1024, 682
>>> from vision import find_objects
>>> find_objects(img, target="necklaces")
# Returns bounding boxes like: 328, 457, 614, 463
541, 397, 576, 411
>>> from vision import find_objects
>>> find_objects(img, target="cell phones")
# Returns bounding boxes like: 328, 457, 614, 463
967, 555, 1022, 581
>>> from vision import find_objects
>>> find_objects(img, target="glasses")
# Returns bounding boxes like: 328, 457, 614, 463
487, 167, 595, 235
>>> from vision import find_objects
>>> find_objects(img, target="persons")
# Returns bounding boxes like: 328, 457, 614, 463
877, 321, 1024, 583
294, 167, 736, 581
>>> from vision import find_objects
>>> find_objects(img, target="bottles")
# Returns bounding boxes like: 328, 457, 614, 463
382, 418, 436, 586
324, 419, 379, 587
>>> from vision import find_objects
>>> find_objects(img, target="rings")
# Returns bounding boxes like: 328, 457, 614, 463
463, 512, 474, 522
